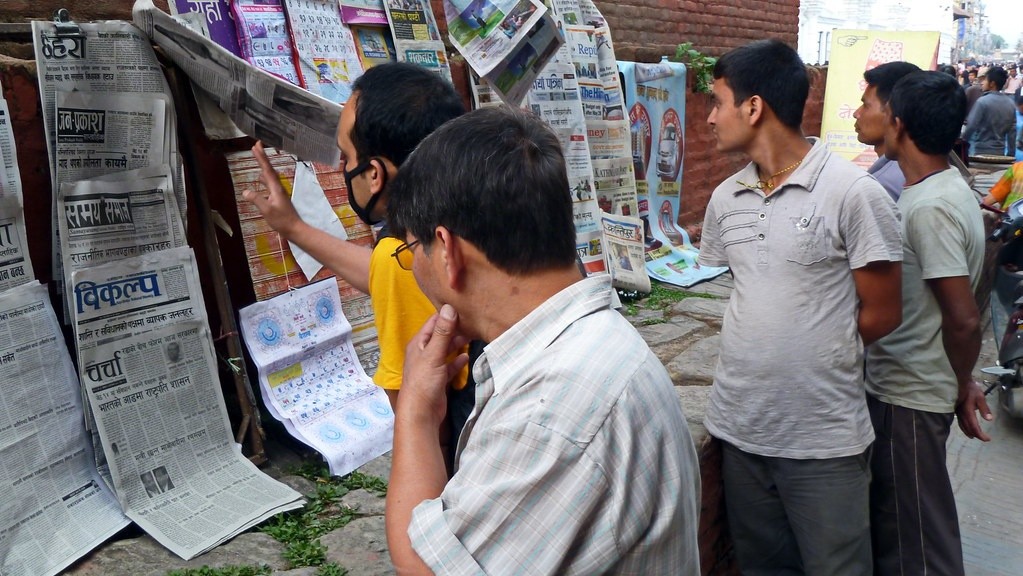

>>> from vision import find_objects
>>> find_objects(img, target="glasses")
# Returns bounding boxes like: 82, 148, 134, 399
391, 225, 450, 271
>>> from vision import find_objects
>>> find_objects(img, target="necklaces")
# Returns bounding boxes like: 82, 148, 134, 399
761, 157, 806, 190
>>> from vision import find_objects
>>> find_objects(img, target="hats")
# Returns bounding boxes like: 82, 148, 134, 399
977, 67, 989, 78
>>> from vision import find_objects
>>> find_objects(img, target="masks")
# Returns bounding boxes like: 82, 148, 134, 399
342, 157, 389, 226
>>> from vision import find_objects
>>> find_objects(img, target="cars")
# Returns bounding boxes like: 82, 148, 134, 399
656, 123, 680, 179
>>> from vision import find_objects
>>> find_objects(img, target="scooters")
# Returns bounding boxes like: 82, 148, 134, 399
970, 187, 1023, 421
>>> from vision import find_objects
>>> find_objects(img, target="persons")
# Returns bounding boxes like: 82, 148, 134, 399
852, 56, 1022, 576
244, 63, 489, 489
696, 40, 904, 576
384, 108, 703, 576
